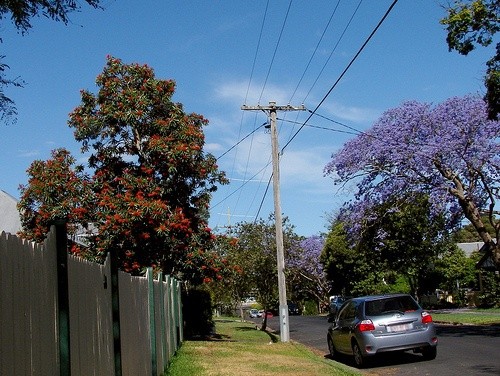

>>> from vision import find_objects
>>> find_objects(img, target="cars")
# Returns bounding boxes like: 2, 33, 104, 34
326, 293, 439, 369
328, 296, 351, 322
249, 309, 274, 318
274, 300, 301, 315
